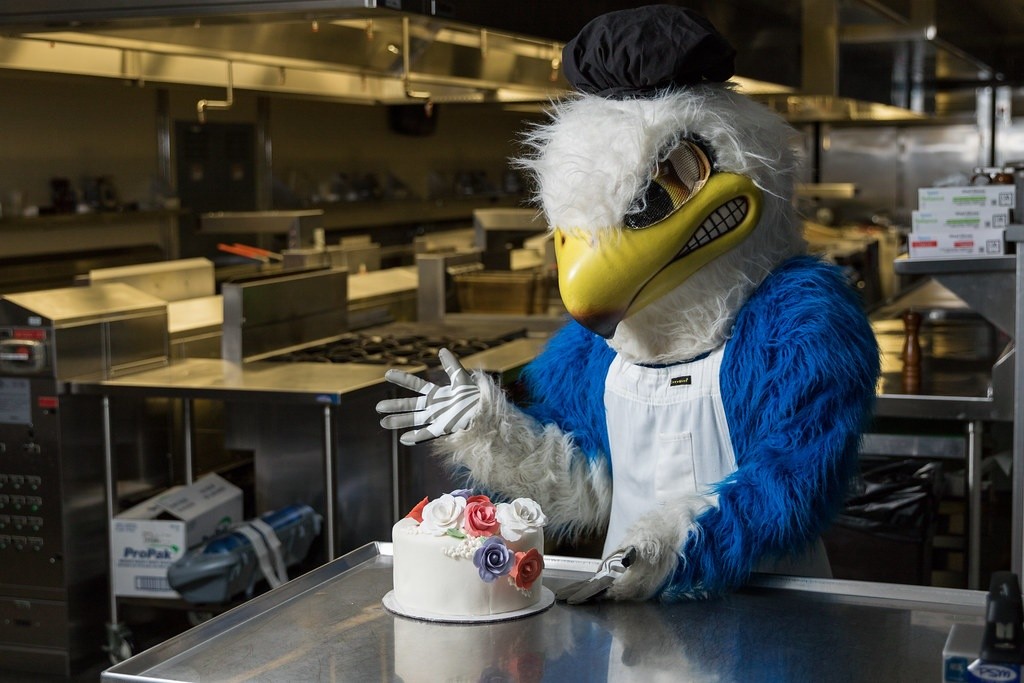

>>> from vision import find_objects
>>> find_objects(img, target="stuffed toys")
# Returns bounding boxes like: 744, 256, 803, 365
375, 6, 880, 606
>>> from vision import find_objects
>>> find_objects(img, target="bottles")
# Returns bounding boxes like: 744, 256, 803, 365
900, 306, 922, 366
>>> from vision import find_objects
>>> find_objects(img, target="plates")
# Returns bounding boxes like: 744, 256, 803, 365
381, 583, 556, 622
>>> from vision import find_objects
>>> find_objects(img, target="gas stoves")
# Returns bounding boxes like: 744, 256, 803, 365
254, 316, 488, 368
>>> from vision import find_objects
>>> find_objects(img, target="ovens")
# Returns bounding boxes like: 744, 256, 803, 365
0, 287, 176, 676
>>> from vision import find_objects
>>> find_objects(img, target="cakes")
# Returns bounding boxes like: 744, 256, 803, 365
393, 487, 548, 615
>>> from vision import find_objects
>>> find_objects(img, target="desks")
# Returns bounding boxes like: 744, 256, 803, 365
102, 518, 1003, 682
63, 352, 431, 670
